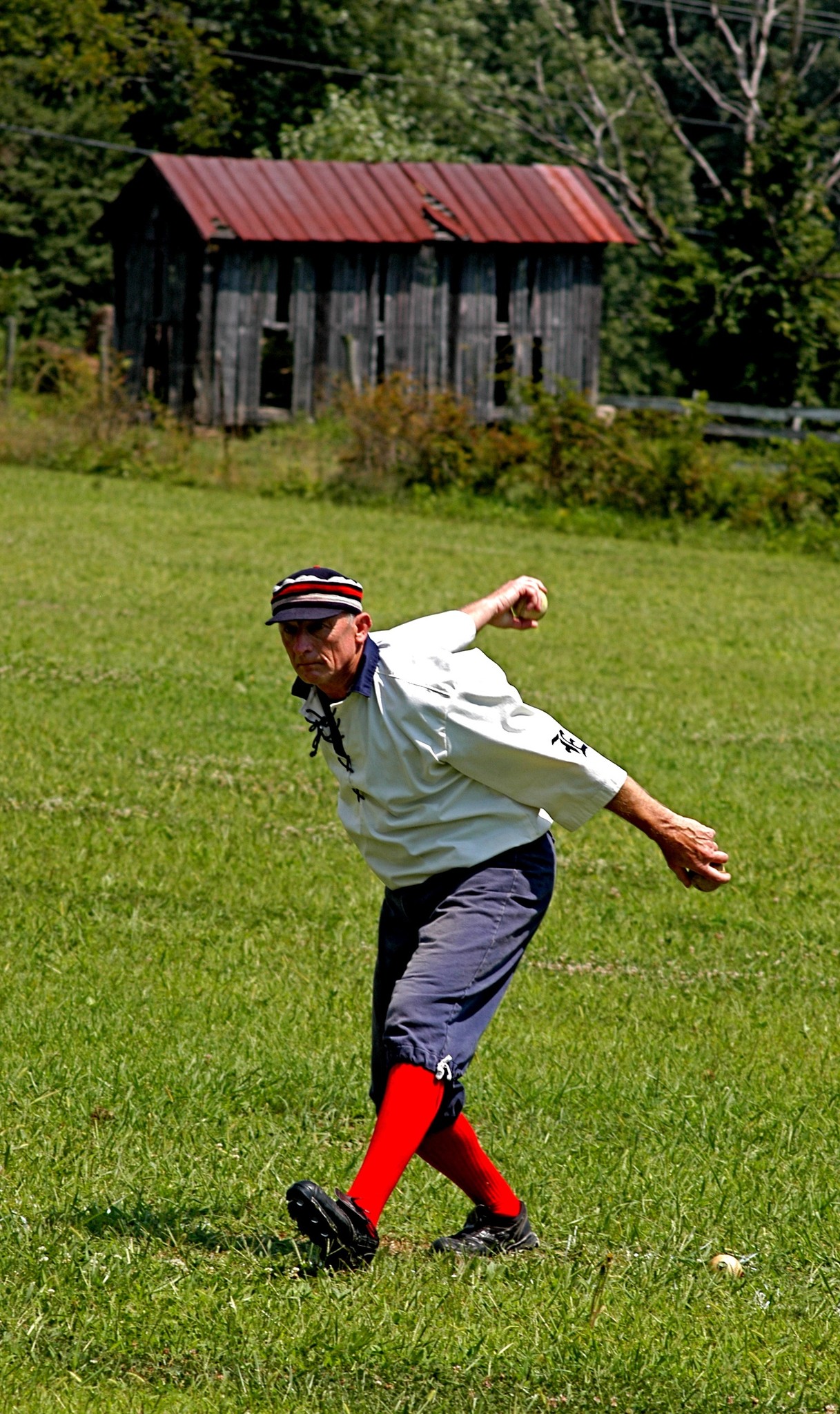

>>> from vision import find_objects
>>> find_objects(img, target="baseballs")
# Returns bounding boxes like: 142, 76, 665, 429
709, 1253, 744, 1279
688, 862, 726, 892
513, 588, 548, 621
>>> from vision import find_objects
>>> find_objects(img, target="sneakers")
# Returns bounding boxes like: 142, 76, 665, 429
427, 1201, 540, 1259
286, 1180, 379, 1271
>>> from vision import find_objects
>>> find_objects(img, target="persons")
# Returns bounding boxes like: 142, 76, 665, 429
259, 564, 732, 1269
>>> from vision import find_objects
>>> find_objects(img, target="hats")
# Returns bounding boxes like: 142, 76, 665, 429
265, 566, 364, 626
